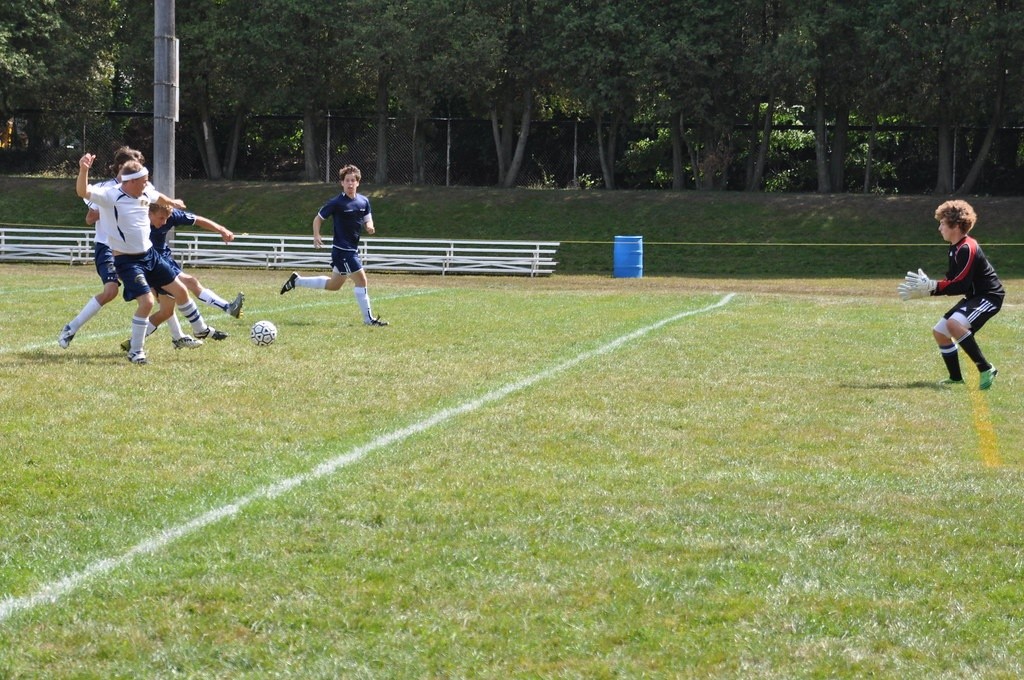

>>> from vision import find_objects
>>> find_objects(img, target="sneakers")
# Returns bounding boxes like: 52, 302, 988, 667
194, 326, 228, 341
364, 314, 389, 327
128, 350, 147, 365
172, 335, 203, 350
121, 339, 131, 351
229, 292, 245, 319
280, 271, 301, 295
979, 363, 998, 391
939, 378, 965, 386
58, 325, 75, 349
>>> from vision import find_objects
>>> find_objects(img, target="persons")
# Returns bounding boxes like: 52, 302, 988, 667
898, 199, 1005, 390
58, 146, 246, 364
280, 164, 390, 326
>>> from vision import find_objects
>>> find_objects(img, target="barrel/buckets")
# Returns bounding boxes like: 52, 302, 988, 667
613, 236, 643, 278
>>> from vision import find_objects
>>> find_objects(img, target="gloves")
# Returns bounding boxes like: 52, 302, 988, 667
897, 284, 931, 302
904, 268, 937, 291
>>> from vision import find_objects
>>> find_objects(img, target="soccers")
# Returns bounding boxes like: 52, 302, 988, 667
250, 319, 278, 348
